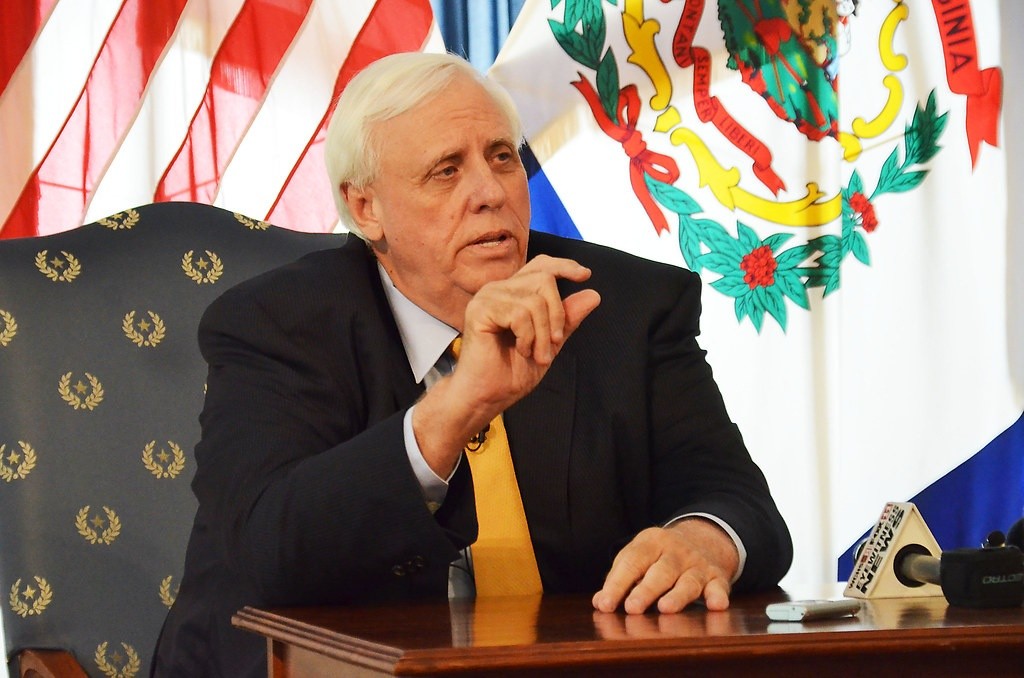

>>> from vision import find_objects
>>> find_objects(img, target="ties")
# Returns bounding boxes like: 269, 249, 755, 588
450, 337, 542, 596
473, 597, 541, 646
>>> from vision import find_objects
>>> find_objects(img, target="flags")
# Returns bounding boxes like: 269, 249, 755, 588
483, 0, 1024, 600
0, 0, 448, 240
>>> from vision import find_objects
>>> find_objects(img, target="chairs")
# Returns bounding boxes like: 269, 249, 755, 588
0, 201, 349, 678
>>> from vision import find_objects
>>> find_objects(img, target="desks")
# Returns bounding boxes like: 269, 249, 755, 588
231, 593, 1024, 678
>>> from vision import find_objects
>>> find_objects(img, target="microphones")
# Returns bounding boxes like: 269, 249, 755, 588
844, 501, 1024, 610
477, 425, 490, 443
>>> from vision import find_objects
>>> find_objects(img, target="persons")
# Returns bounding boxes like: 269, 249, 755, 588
145, 52, 795, 678
